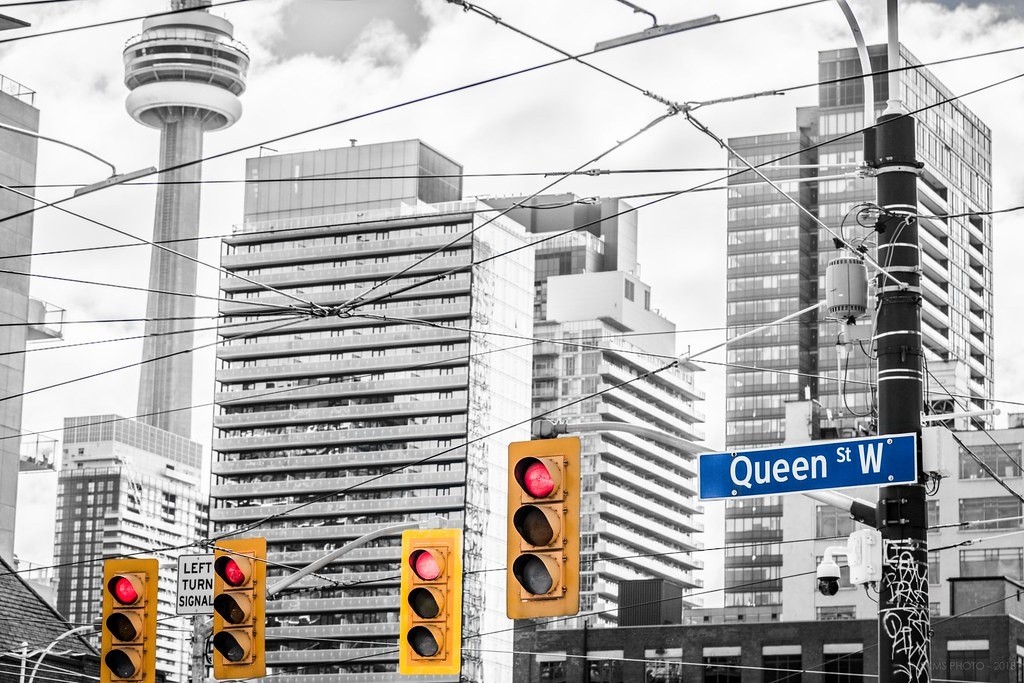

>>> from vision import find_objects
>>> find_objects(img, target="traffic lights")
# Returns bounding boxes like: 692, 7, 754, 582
408, 546, 448, 659
512, 456, 566, 602
106, 572, 145, 679
212, 555, 256, 666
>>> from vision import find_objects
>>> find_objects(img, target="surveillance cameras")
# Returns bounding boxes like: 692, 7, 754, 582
817, 564, 840, 596
